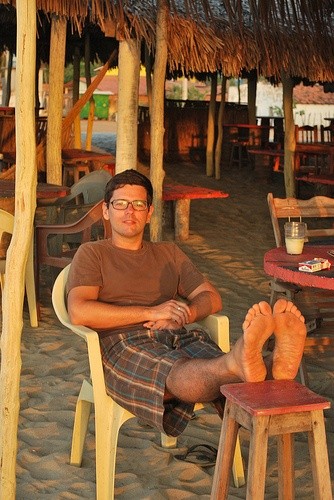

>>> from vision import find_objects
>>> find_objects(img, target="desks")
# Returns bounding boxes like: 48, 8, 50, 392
62, 146, 110, 204
0, 178, 72, 312
225, 122, 275, 167
297, 141, 334, 178
262, 242, 334, 388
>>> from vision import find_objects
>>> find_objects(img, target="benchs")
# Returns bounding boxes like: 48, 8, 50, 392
162, 179, 229, 240
246, 148, 283, 184
296, 174, 334, 198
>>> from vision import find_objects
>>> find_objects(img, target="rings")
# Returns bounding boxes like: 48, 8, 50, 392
178, 306, 181, 311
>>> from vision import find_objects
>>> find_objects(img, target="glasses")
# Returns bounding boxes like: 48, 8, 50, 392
109, 198, 150, 210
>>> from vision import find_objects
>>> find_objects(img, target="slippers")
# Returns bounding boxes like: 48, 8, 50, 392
173, 444, 218, 466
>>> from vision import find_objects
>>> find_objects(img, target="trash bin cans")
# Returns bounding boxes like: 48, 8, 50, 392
78, 91, 114, 120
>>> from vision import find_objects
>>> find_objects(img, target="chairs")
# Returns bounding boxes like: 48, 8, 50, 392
320, 125, 334, 174
33, 197, 112, 322
295, 124, 318, 173
266, 191, 334, 352
51, 262, 246, 500
39, 170, 112, 283
0, 209, 40, 328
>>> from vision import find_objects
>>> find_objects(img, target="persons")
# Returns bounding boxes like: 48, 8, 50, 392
66, 169, 307, 437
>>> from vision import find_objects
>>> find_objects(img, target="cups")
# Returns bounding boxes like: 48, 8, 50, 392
284, 222, 307, 254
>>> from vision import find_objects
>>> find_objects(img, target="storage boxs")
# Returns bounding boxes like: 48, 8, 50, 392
298, 257, 331, 273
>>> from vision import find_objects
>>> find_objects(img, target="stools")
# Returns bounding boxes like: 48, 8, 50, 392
209, 379, 334, 500
228, 134, 252, 171
